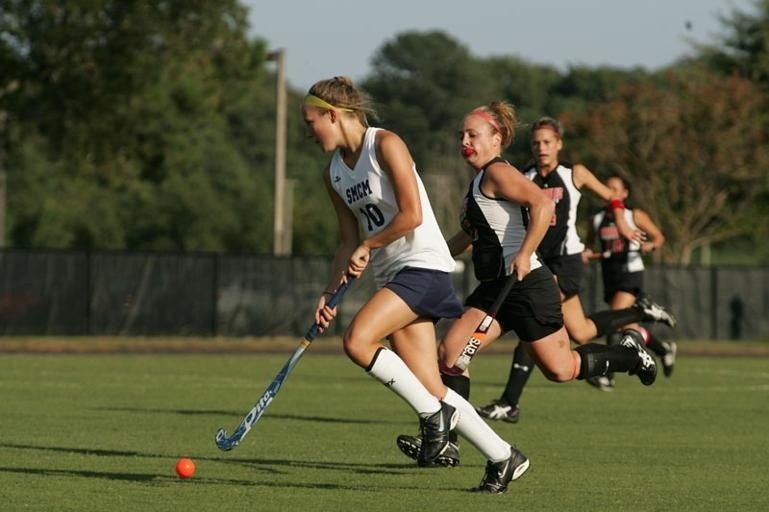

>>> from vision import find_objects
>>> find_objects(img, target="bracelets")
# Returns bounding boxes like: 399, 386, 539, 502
651, 241, 658, 251
321, 289, 337, 297
610, 199, 626, 213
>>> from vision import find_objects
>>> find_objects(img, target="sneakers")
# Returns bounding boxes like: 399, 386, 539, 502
474, 398, 520, 424
661, 339, 677, 378
616, 327, 658, 386
470, 446, 531, 495
632, 293, 679, 329
418, 400, 460, 468
585, 373, 616, 392
397, 435, 461, 467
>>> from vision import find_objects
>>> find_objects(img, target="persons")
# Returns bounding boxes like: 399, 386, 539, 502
582, 173, 679, 395
471, 114, 678, 428
392, 97, 660, 471
297, 70, 532, 495
